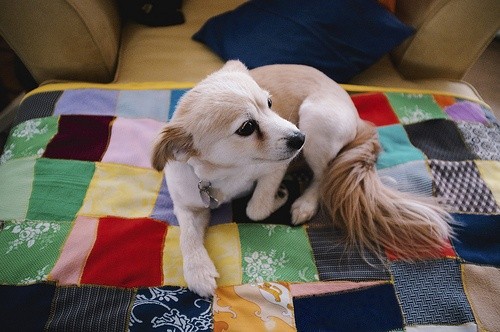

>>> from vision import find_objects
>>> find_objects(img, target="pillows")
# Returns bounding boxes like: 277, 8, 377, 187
192, 0, 418, 82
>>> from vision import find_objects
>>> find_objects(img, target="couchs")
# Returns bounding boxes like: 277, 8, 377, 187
0, 0, 500, 101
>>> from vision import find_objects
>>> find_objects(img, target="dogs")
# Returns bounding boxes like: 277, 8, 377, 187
151, 59, 467, 298
